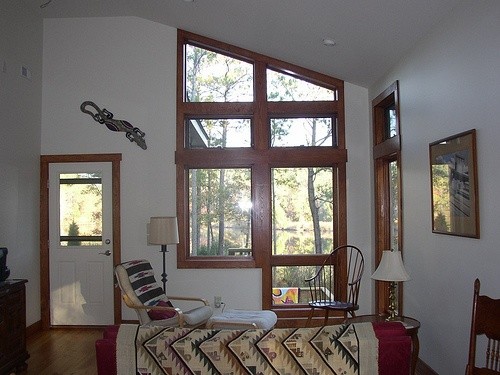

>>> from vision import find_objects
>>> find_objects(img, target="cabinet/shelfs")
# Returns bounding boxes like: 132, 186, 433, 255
0, 279, 31, 375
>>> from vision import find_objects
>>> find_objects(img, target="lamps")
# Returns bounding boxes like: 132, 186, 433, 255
148, 216, 180, 295
371, 249, 410, 323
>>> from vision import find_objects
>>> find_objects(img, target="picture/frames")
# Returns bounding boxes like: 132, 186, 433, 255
427, 128, 480, 240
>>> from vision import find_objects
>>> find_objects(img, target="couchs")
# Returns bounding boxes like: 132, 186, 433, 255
94, 324, 414, 374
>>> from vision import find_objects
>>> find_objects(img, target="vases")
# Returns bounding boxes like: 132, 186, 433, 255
0, 248, 10, 283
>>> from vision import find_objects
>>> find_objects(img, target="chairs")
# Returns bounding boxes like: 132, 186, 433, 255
302, 246, 364, 328
114, 257, 278, 330
465, 278, 499, 375
